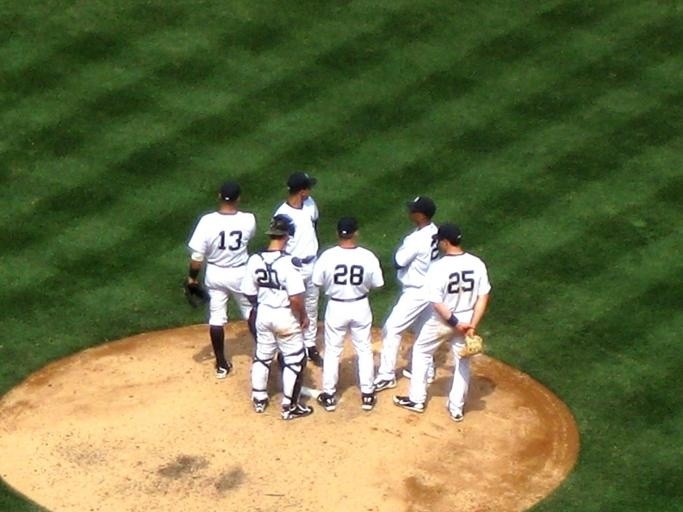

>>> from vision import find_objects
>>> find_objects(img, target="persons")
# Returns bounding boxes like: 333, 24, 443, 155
270, 173, 323, 367
184, 181, 259, 377
393, 223, 492, 421
373, 195, 442, 393
313, 218, 385, 411
242, 213, 315, 419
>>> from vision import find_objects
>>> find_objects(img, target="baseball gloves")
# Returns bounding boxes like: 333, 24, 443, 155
456, 334, 485, 359
183, 275, 212, 308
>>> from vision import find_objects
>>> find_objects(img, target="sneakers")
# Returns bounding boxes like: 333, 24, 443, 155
252, 397, 270, 413
316, 393, 337, 412
393, 395, 425, 413
374, 378, 397, 392
281, 403, 313, 420
401, 366, 435, 384
305, 346, 323, 368
214, 362, 232, 380
445, 400, 465, 422
361, 393, 377, 411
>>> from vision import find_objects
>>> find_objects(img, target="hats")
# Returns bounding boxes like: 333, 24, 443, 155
221, 181, 241, 201
406, 196, 436, 217
286, 171, 316, 191
336, 216, 359, 234
264, 214, 296, 238
431, 223, 463, 243
